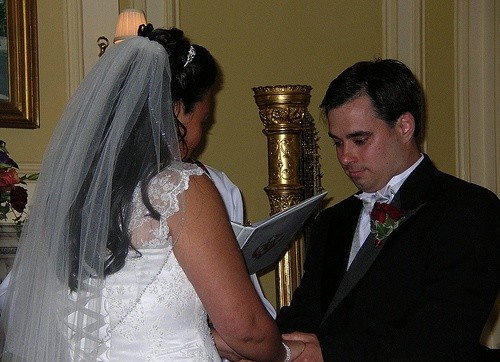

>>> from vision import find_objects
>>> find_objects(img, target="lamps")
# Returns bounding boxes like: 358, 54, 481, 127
97, 8, 147, 61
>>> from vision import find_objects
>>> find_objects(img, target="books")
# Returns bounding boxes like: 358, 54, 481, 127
227, 191, 329, 275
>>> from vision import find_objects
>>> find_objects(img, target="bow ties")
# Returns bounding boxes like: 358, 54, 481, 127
353, 185, 395, 214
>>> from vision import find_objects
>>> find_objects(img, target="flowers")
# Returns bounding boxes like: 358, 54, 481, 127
368, 195, 429, 247
0, 139, 40, 240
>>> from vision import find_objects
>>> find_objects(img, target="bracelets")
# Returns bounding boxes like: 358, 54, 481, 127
282, 342, 291, 362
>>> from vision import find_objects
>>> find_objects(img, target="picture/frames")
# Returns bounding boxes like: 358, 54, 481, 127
0, 0, 40, 130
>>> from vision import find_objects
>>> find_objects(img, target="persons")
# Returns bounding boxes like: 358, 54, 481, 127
272, 58, 500, 362
0, 23, 306, 362
191, 159, 276, 321
